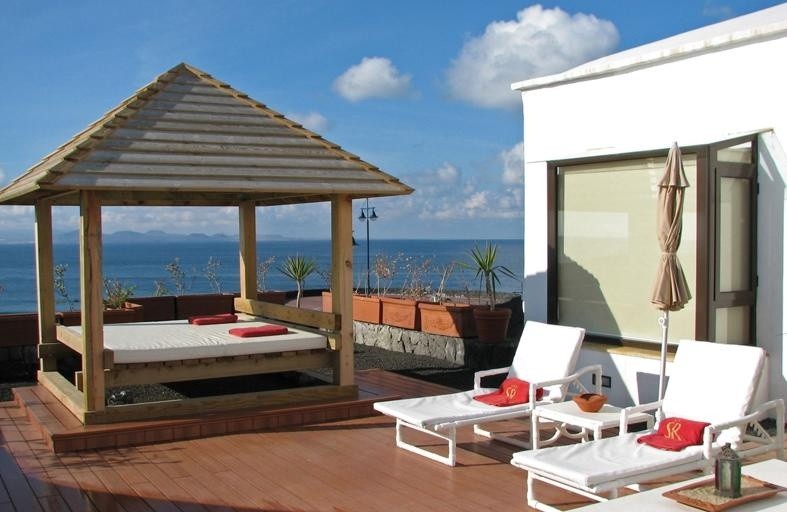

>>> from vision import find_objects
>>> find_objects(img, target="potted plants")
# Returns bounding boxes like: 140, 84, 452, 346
323, 240, 521, 346
102, 279, 144, 322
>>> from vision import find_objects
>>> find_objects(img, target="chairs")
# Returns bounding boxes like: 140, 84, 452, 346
374, 320, 602, 466
509, 340, 785, 512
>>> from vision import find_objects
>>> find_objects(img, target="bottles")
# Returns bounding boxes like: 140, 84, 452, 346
713, 443, 746, 499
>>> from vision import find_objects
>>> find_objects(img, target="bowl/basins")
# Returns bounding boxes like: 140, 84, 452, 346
573, 393, 609, 413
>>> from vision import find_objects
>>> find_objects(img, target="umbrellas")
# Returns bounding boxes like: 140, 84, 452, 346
650, 143, 692, 429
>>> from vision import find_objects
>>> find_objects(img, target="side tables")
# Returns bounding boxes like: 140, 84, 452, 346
532, 400, 654, 450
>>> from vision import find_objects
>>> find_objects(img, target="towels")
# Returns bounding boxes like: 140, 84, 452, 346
188, 313, 238, 325
637, 419, 715, 451
473, 378, 543, 406
229, 325, 288, 337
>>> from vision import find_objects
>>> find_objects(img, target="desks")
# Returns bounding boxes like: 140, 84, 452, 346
563, 459, 787, 511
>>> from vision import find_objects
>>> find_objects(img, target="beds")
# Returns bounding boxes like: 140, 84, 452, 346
67, 313, 327, 364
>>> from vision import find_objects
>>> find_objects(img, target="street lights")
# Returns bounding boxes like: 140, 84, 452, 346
358, 205, 379, 296
